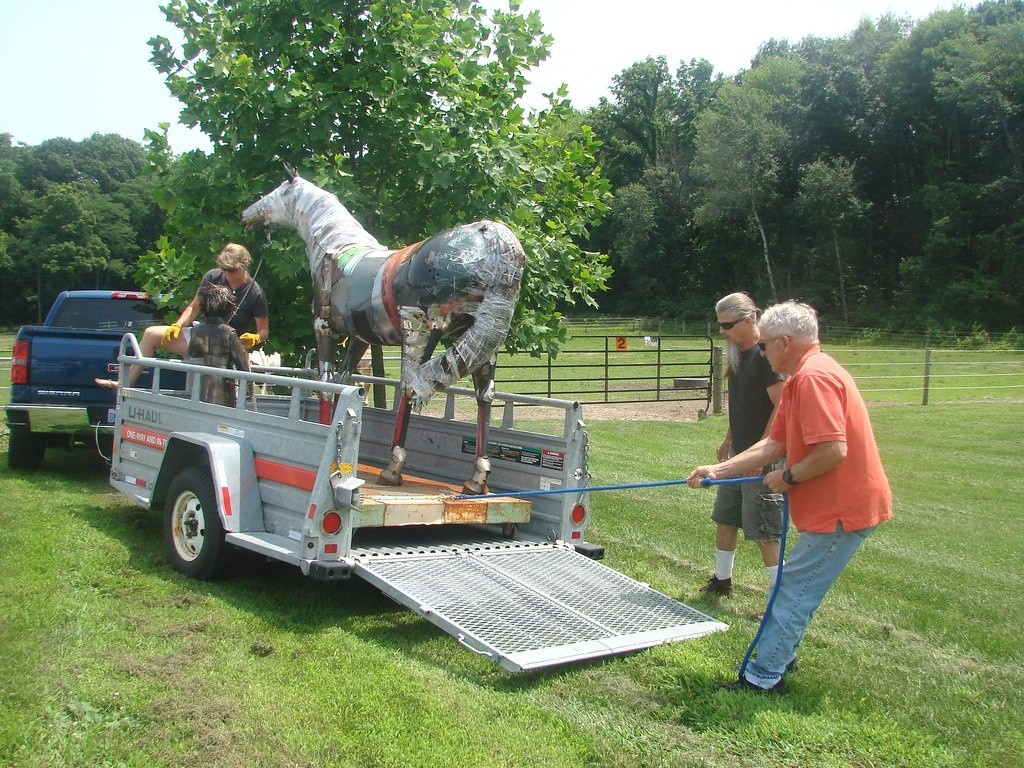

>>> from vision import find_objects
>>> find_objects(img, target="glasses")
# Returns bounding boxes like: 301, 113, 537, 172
717, 317, 748, 330
758, 335, 793, 351
221, 267, 238, 272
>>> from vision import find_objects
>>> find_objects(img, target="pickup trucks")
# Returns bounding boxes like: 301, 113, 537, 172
6, 292, 200, 469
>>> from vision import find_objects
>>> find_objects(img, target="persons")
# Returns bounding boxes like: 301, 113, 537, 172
93, 243, 270, 395
685, 293, 787, 597
187, 280, 254, 408
688, 301, 891, 695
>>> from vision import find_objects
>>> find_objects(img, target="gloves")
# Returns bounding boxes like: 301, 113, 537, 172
160, 324, 182, 346
239, 332, 261, 350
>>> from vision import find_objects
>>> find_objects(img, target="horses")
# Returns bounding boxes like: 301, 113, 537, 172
241, 160, 525, 496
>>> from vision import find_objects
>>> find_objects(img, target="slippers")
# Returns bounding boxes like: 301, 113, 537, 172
95, 378, 117, 393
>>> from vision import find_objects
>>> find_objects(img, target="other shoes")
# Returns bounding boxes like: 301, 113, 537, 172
716, 678, 786, 696
734, 657, 798, 675
686, 573, 734, 596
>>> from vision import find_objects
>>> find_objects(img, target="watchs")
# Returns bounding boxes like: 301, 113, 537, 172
783, 468, 800, 486
